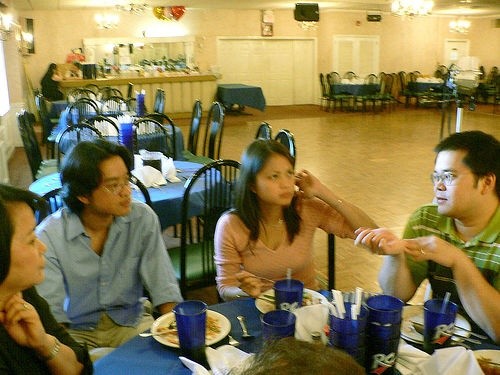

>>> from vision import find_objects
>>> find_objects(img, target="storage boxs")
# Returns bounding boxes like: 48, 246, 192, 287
83, 64, 96, 80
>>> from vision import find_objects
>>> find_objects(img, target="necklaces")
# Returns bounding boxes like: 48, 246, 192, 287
266, 218, 284, 225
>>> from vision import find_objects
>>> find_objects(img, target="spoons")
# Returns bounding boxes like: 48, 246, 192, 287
227, 333, 240, 346
237, 316, 255, 338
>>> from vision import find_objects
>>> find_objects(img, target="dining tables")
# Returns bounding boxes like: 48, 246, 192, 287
52, 124, 183, 157
457, 79, 496, 104
57, 108, 125, 123
88, 293, 427, 375
333, 79, 381, 112
51, 100, 68, 118
408, 81, 446, 109
29, 159, 222, 236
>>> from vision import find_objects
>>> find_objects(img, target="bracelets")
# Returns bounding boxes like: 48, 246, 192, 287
36, 337, 60, 361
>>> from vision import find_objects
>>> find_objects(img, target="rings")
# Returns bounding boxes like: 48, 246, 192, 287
421, 248, 425, 254
23, 302, 27, 308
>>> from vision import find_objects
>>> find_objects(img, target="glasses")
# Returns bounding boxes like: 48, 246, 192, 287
98, 174, 139, 193
430, 172, 485, 186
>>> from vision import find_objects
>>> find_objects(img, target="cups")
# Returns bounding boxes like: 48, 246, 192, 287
119, 123, 133, 151
423, 298, 457, 355
273, 280, 305, 311
261, 310, 296, 346
137, 93, 145, 119
173, 300, 207, 351
327, 295, 404, 375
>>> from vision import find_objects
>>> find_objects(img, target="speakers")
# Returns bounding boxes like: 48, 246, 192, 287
296, 3, 320, 21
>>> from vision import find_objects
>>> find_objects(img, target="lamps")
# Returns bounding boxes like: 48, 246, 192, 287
93, 13, 120, 31
0, 12, 34, 56
449, 15, 472, 35
390, 0, 435, 22
115, 2, 149, 15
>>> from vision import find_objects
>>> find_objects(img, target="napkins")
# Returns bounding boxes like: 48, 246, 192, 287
293, 305, 332, 346
178, 344, 258, 375
129, 165, 167, 192
396, 338, 485, 375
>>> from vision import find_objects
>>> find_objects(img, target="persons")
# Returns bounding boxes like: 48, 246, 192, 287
31, 139, 184, 354
41, 62, 64, 101
476, 66, 488, 103
214, 140, 377, 299
354, 130, 500, 346
65, 62, 83, 80
0, 184, 92, 375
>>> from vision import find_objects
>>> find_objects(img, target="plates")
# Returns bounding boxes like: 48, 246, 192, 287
400, 304, 471, 348
471, 349, 500, 375
150, 309, 231, 348
255, 289, 328, 314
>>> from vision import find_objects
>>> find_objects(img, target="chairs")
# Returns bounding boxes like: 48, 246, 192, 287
0, 64, 500, 304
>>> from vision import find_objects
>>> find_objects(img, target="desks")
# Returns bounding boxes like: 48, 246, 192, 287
217, 83, 266, 116
59, 72, 222, 120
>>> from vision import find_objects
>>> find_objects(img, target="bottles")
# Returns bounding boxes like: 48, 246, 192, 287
310, 331, 323, 345
